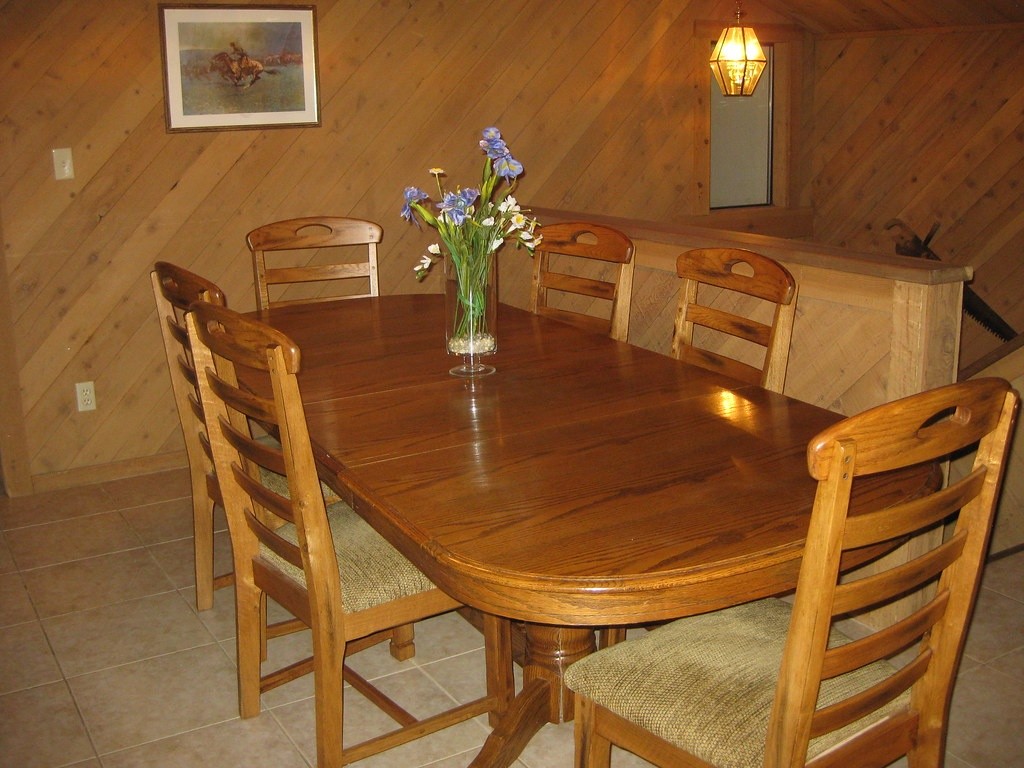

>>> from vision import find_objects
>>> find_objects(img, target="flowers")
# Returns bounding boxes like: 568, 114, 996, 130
400, 126, 543, 342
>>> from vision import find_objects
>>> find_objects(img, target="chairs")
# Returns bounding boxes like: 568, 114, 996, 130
529, 222, 799, 651
148, 261, 515, 768
245, 217, 383, 311
560, 376, 1022, 768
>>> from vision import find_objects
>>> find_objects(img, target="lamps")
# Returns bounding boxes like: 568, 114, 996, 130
709, 8, 768, 98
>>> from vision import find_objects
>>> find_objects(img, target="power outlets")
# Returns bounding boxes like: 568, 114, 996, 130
75, 381, 98, 413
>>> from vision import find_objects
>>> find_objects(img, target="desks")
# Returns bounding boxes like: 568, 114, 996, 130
232, 292, 946, 768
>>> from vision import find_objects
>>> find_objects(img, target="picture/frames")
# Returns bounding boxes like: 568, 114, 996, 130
158, 2, 322, 135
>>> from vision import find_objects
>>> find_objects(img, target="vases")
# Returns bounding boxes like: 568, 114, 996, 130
444, 251, 499, 378
